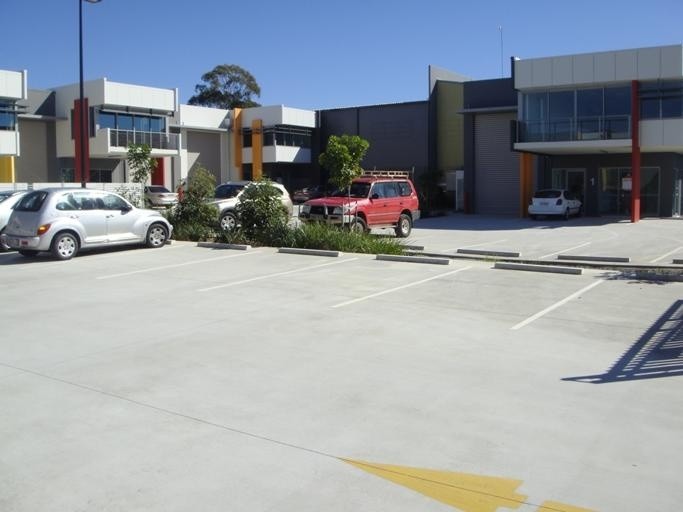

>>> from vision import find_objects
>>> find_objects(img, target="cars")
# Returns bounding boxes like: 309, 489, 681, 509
1, 188, 174, 261
527, 189, 582, 220
143, 186, 180, 209
293, 185, 320, 202
201, 181, 295, 233
0, 190, 32, 252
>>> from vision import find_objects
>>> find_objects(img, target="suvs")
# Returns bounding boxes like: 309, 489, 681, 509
299, 170, 421, 238
